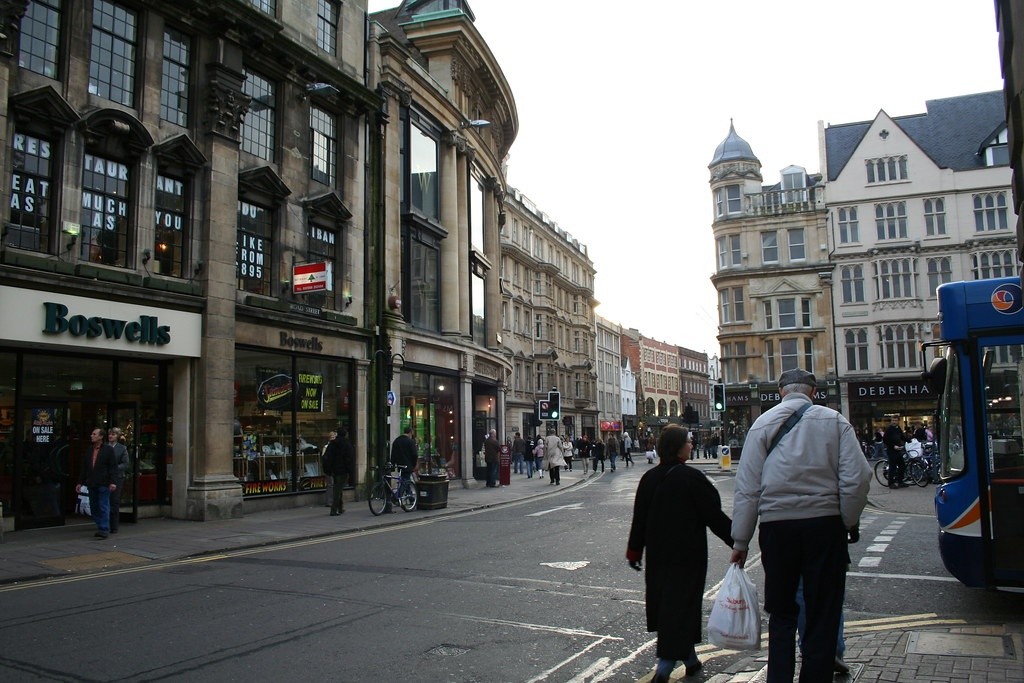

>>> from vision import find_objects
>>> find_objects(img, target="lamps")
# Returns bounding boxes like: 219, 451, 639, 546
194, 260, 202, 276
64, 230, 79, 250
455, 116, 490, 134
143, 248, 152, 266
346, 295, 352, 306
297, 81, 341, 102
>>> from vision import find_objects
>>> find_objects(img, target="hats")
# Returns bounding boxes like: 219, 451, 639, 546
778, 368, 817, 387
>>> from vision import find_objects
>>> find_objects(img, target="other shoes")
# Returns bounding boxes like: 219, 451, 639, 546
798, 648, 803, 657
889, 483, 899, 489
650, 674, 668, 683
110, 530, 117, 533
94, 532, 108, 538
330, 512, 340, 516
686, 661, 702, 676
488, 458, 635, 488
833, 655, 849, 673
898, 483, 909, 487
339, 509, 345, 514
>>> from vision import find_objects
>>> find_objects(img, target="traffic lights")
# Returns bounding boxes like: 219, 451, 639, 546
713, 383, 726, 412
538, 391, 561, 422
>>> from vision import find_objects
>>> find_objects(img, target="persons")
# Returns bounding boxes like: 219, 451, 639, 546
701, 432, 719, 460
484, 429, 504, 488
322, 427, 354, 516
391, 427, 418, 507
950, 412, 1020, 454
107, 426, 129, 534
729, 367, 874, 683
507, 431, 545, 479
75, 428, 118, 541
626, 423, 734, 683
854, 415, 934, 489
566, 431, 656, 475
545, 428, 569, 485
689, 436, 697, 461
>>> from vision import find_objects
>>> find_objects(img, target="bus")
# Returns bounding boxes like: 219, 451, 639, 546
916, 275, 1024, 590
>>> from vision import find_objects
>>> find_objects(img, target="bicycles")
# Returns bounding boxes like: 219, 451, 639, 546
366, 462, 418, 517
873, 440, 940, 488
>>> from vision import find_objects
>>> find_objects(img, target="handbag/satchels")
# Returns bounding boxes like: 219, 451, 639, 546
905, 438, 924, 458
74, 486, 92, 517
706, 561, 762, 650
646, 452, 655, 460
653, 450, 656, 458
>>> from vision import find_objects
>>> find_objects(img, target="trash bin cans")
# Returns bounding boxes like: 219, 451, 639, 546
415, 473, 449, 509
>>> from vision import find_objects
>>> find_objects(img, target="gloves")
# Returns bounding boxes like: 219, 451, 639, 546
847, 528, 859, 543
